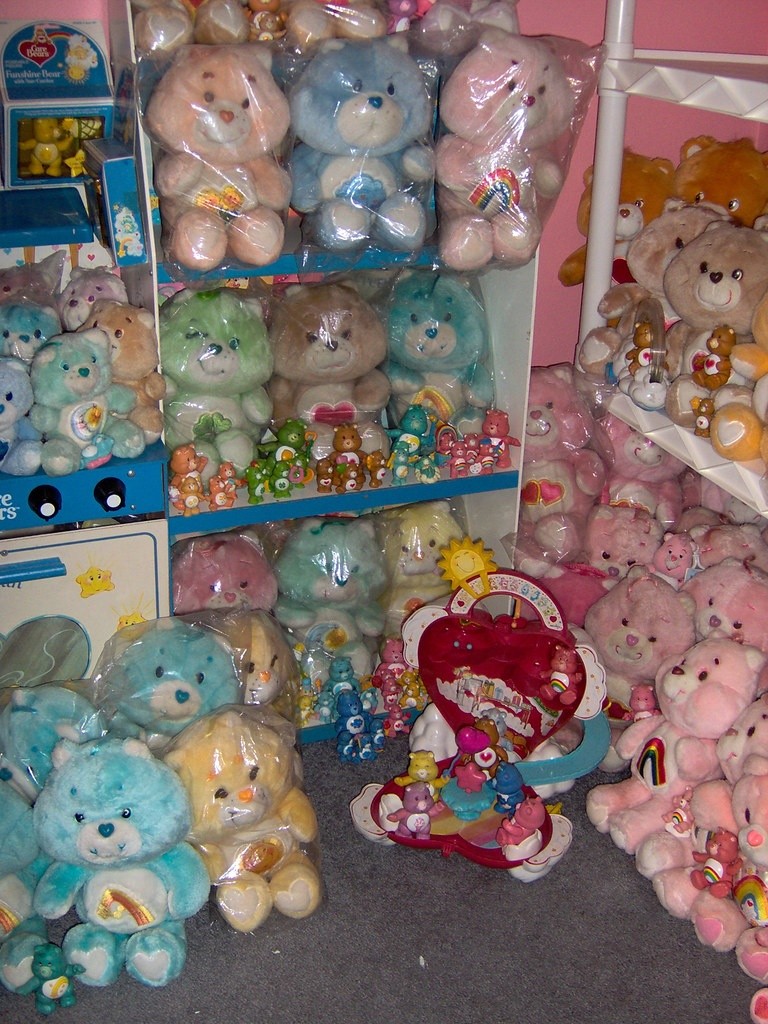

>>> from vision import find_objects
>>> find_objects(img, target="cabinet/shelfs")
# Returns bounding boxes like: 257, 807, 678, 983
111, 0, 539, 748
570, 0, 768, 523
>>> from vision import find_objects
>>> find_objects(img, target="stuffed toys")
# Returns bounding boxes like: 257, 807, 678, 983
32, 737, 211, 988
0, 0, 768, 1024
